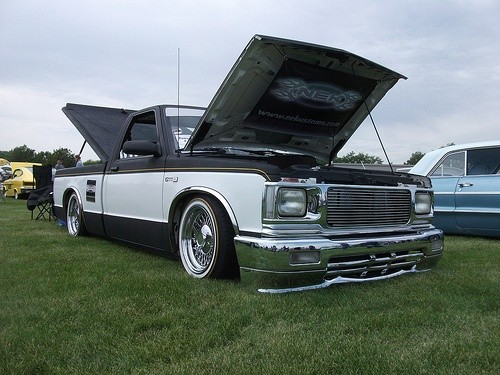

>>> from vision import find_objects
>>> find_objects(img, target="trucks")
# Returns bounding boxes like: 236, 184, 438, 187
3, 162, 42, 200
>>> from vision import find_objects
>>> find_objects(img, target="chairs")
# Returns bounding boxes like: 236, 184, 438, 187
29, 164, 56, 222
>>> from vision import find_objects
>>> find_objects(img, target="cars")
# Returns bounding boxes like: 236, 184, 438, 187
50, 33, 447, 295
399, 141, 500, 238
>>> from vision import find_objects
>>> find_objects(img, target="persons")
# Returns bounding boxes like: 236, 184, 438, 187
55, 158, 65, 170
75, 155, 84, 167
